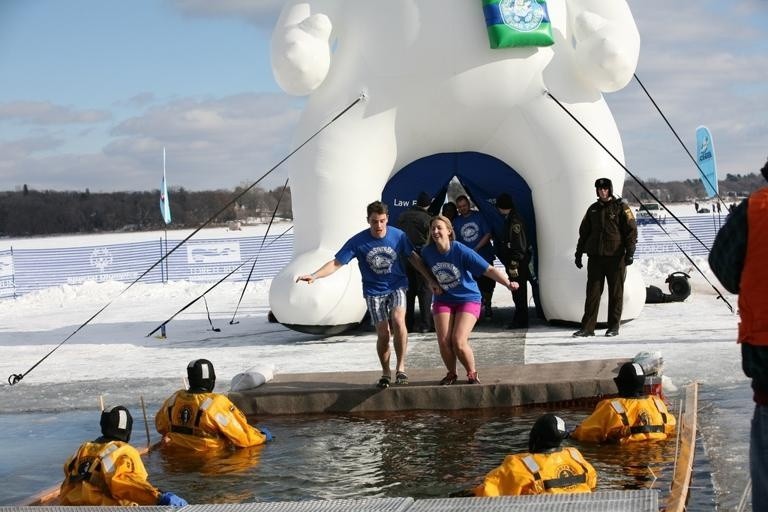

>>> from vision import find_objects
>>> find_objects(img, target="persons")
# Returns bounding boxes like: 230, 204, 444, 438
572, 177, 639, 339
294, 200, 438, 384
395, 190, 535, 334
567, 362, 676, 446
707, 159, 768, 510
424, 216, 521, 383
61, 400, 188, 505
438, 414, 599, 498
712, 204, 715, 212
694, 202, 699, 213
716, 202, 720, 212
152, 357, 277, 467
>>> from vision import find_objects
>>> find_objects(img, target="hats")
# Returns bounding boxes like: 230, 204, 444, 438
595, 178, 613, 197
613, 362, 646, 397
496, 194, 512, 209
528, 414, 567, 453
188, 359, 215, 392
101, 405, 133, 443
417, 191, 431, 207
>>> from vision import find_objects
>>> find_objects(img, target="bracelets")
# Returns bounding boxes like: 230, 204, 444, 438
311, 272, 320, 282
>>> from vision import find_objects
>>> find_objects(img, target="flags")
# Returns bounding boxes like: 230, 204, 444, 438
159, 143, 173, 224
695, 127, 719, 199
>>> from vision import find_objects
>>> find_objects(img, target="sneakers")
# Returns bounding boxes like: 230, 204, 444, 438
465, 372, 480, 384
573, 329, 595, 337
440, 372, 458, 385
503, 322, 519, 329
395, 371, 408, 385
606, 329, 618, 336
378, 375, 391, 388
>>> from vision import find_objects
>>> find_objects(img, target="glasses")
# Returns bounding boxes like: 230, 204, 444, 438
598, 186, 608, 190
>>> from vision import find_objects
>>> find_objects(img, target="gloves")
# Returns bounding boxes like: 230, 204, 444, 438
576, 255, 583, 268
159, 492, 189, 506
624, 254, 633, 265
260, 428, 272, 441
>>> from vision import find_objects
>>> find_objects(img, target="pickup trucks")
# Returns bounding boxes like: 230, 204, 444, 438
636, 203, 666, 225
